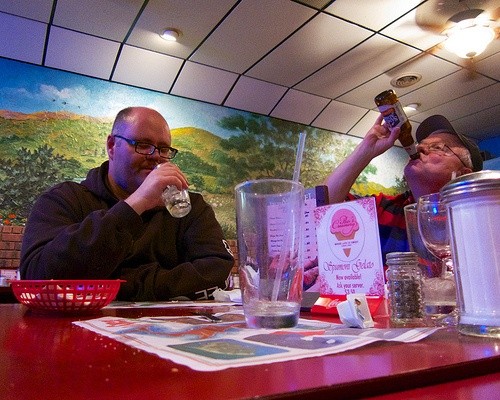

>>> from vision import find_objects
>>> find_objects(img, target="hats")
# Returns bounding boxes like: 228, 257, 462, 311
415, 115, 483, 172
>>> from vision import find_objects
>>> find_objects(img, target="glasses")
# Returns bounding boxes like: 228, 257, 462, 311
416, 142, 468, 168
114, 134, 178, 159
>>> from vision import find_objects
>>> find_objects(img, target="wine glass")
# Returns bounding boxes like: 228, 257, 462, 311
417, 193, 460, 326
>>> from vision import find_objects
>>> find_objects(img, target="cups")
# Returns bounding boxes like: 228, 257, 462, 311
235, 179, 304, 330
404, 201, 457, 328
152, 162, 192, 218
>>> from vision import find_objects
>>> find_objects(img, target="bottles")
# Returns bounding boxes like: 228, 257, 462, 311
440, 170, 500, 340
374, 89, 420, 163
385, 252, 425, 325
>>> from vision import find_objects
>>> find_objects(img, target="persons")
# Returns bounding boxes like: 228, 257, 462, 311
21, 107, 235, 299
321, 110, 485, 271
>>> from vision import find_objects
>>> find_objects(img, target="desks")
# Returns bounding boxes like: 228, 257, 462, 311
0, 300, 500, 400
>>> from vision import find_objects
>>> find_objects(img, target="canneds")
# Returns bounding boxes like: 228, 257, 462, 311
386, 251, 425, 325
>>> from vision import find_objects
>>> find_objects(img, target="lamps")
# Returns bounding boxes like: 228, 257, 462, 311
440, 0, 495, 59
159, 27, 184, 42
406, 103, 422, 110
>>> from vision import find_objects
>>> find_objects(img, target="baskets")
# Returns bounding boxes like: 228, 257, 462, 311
6, 280, 127, 315
